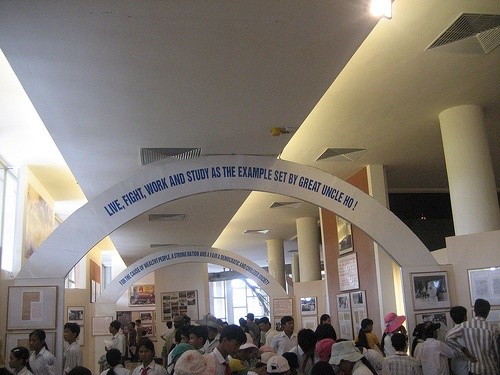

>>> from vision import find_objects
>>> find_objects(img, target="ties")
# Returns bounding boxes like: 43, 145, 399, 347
222, 362, 231, 375
141, 367, 150, 375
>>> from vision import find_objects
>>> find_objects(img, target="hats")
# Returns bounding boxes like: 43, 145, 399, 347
171, 315, 185, 322
258, 352, 290, 372
421, 321, 441, 333
328, 340, 364, 365
384, 312, 406, 333
173, 350, 216, 375
316, 338, 336, 361
167, 344, 195, 367
239, 332, 259, 351
245, 313, 254, 317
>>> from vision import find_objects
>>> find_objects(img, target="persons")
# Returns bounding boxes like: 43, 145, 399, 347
411, 306, 468, 375
105, 320, 125, 369
445, 299, 500, 375
28, 329, 56, 375
63, 322, 83, 375
161, 312, 419, 375
8, 346, 34, 375
0, 368, 14, 375
132, 341, 168, 375
100, 349, 132, 375
119, 319, 148, 363
66, 366, 91, 375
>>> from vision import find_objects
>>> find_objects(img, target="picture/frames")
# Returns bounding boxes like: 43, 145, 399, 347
408, 271, 451, 311
139, 324, 155, 338
336, 293, 354, 341
350, 290, 369, 343
128, 284, 156, 307
472, 309, 500, 322
412, 310, 455, 342
140, 311, 156, 324
6, 286, 58, 331
301, 315, 319, 332
76, 325, 85, 346
5, 332, 56, 368
66, 306, 85, 327
272, 297, 292, 318
467, 265, 500, 306
273, 318, 283, 332
91, 315, 114, 337
300, 296, 318, 316
